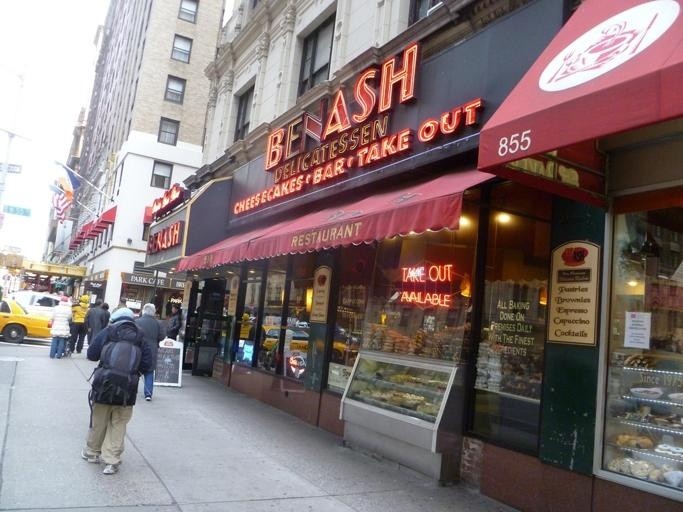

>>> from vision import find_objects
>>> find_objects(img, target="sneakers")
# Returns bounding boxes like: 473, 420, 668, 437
79, 445, 100, 464
100, 462, 121, 475
144, 396, 151, 401
141, 302, 157, 317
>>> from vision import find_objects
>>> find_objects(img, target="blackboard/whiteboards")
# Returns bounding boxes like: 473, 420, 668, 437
152, 338, 183, 388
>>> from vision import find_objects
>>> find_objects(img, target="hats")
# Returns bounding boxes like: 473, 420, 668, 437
79, 294, 89, 303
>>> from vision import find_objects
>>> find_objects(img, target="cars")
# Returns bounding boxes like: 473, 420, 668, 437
222, 312, 359, 363
1, 290, 74, 326
0, 295, 53, 345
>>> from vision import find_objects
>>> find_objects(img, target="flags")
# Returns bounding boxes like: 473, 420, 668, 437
51, 162, 82, 224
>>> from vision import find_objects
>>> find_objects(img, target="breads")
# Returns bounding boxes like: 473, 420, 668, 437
624, 354, 654, 369
607, 406, 683, 481
383, 330, 410, 354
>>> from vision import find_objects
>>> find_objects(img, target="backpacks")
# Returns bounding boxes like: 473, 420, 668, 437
84, 320, 147, 407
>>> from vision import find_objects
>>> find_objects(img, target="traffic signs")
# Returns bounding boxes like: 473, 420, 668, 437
3, 205, 32, 217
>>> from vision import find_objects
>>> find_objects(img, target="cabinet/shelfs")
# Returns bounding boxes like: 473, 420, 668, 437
339, 348, 465, 482
602, 349, 683, 491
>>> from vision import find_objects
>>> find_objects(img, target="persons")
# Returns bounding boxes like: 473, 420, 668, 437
47, 294, 181, 401
81, 307, 152, 476
